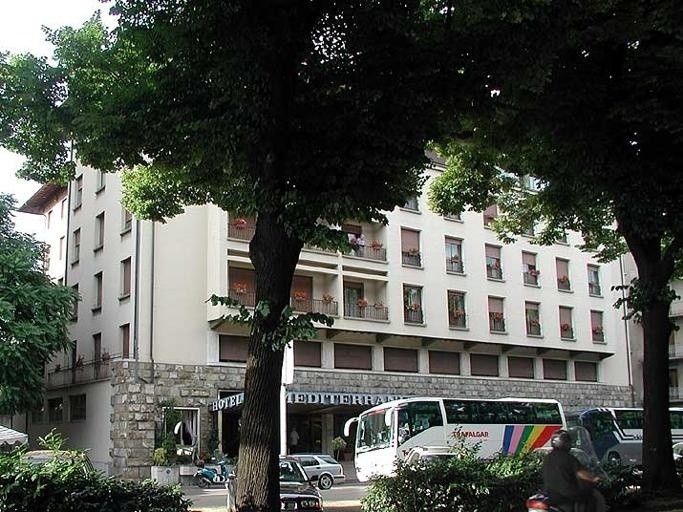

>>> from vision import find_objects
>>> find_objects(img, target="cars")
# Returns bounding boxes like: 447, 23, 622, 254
21, 447, 99, 481
672, 442, 683, 474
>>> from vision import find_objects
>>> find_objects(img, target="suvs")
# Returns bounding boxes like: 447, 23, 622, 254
225, 454, 325, 512
280, 453, 347, 492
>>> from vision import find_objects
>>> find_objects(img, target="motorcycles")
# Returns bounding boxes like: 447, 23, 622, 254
194, 454, 231, 489
527, 426, 608, 512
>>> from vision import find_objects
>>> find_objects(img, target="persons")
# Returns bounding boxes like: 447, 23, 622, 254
288, 425, 300, 453
543, 429, 607, 510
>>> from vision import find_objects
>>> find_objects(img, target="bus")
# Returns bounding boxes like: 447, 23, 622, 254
564, 404, 683, 473
342, 394, 568, 486
